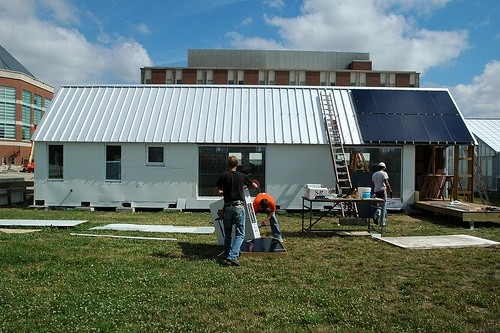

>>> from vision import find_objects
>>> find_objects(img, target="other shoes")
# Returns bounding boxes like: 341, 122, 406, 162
382, 223, 385, 226
227, 257, 240, 265
373, 216, 378, 225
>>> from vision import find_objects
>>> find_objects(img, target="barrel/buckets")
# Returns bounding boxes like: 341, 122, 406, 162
358, 187, 371, 198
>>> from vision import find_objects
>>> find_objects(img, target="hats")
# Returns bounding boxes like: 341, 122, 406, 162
379, 162, 386, 168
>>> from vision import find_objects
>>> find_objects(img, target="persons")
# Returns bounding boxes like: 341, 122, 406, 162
253, 193, 283, 242
372, 162, 393, 227
215, 156, 257, 266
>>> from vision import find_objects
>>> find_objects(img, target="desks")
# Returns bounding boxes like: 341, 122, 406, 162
424, 174, 467, 201
302, 195, 385, 237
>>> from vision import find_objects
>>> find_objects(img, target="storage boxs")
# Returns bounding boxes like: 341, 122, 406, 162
304, 187, 329, 199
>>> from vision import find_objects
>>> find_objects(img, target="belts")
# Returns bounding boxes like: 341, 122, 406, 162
223, 202, 242, 206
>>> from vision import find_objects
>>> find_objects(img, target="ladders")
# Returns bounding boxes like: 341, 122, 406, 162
320, 91, 359, 220
464, 144, 490, 205
